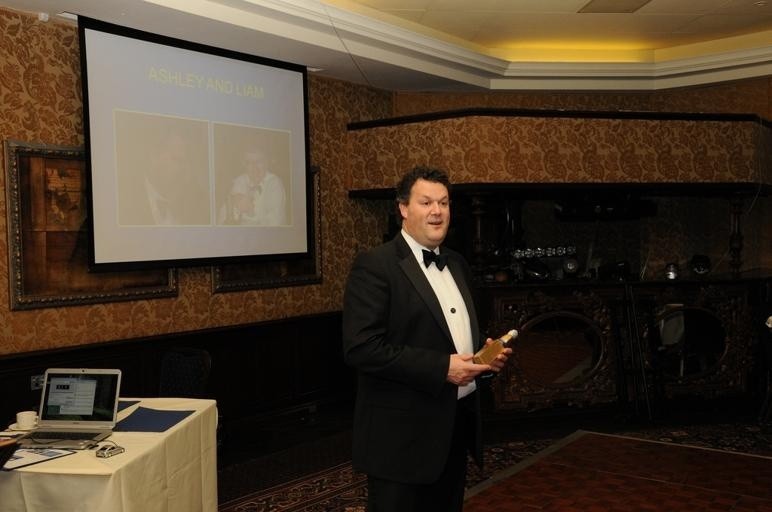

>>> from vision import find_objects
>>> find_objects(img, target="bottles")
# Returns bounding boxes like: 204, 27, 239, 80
472, 329, 519, 366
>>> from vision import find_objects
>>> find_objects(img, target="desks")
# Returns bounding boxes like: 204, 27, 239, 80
1, 397, 218, 512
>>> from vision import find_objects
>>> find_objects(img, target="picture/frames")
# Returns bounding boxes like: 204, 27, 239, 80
114, 108, 212, 231
209, 163, 323, 295
213, 121, 292, 227
3, 139, 180, 312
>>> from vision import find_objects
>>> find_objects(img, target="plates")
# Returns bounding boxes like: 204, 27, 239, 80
9, 422, 34, 431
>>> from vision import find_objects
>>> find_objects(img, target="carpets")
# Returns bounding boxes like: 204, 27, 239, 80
463, 430, 771, 512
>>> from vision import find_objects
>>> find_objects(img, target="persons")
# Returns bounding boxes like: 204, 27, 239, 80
219, 140, 290, 228
340, 165, 514, 511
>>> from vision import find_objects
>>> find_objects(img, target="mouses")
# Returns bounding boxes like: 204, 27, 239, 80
96, 445, 125, 458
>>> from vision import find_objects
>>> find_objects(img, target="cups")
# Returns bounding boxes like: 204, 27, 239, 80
16, 411, 39, 428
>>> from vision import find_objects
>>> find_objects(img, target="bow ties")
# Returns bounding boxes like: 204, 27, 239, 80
422, 249, 449, 271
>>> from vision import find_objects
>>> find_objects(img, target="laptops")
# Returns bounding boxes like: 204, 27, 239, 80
17, 367, 122, 450
